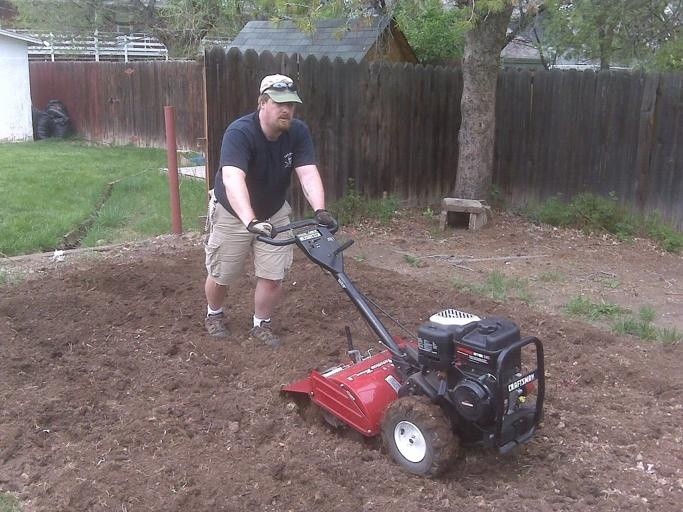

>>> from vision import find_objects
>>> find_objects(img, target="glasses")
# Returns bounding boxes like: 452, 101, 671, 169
261, 82, 297, 94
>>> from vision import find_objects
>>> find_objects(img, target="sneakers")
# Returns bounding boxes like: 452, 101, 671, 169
250, 320, 279, 350
205, 312, 228, 337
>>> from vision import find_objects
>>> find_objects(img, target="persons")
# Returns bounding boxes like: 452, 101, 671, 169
202, 74, 339, 348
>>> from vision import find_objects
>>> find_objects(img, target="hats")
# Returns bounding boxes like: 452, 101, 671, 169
258, 73, 303, 104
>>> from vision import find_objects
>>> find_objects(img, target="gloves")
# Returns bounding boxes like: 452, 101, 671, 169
314, 209, 338, 232
245, 218, 277, 239
